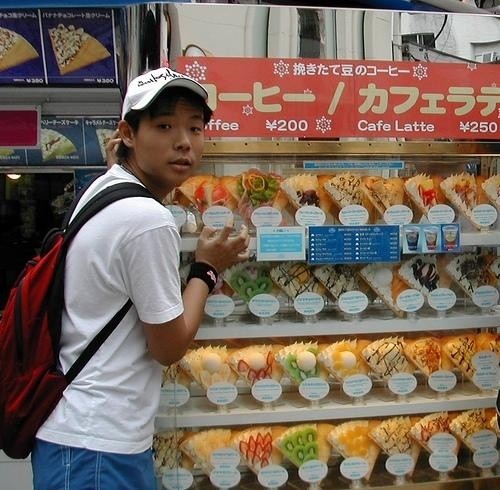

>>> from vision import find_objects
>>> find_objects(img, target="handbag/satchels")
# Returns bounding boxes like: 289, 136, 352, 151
186, 261, 219, 295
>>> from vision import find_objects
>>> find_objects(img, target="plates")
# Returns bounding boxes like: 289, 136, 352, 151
150, 139, 500, 490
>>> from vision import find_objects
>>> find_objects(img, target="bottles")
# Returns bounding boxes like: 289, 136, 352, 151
117, 65, 209, 121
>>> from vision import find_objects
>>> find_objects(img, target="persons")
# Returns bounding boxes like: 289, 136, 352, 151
24, 63, 254, 489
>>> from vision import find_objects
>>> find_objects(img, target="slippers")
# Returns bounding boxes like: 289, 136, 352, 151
1, 223, 68, 461
